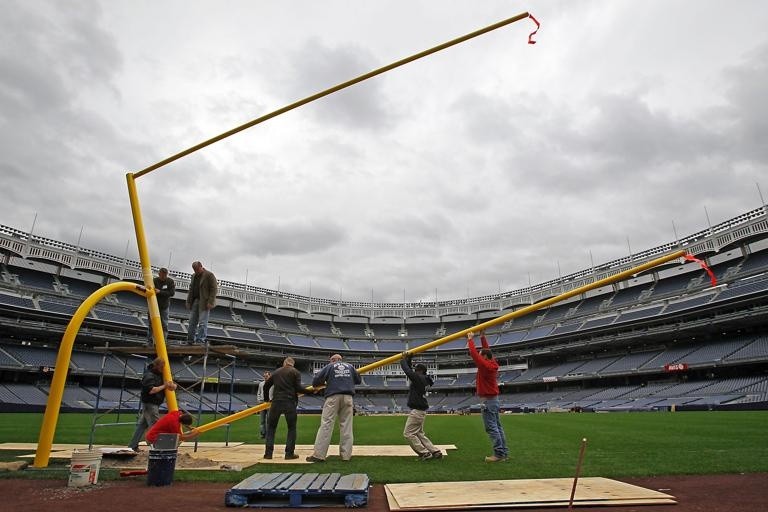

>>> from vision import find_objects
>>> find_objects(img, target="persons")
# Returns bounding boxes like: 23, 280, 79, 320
353, 403, 365, 416
306, 353, 361, 463
128, 357, 178, 452
468, 330, 508, 461
401, 352, 442, 461
182, 261, 217, 347
451, 407, 471, 415
263, 357, 318, 459
257, 371, 276, 439
145, 410, 199, 472
141, 268, 175, 347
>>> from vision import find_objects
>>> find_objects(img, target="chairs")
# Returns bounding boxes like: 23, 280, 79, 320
0, 231, 766, 417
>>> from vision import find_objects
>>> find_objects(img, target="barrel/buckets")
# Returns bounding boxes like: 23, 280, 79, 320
67, 449, 103, 488
145, 448, 178, 487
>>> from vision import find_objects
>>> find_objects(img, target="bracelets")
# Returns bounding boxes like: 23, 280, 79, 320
188, 434, 193, 439
159, 386, 163, 391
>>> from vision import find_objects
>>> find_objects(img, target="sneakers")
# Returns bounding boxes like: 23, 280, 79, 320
306, 456, 324, 462
264, 454, 272, 458
285, 452, 299, 459
416, 451, 442, 461
485, 456, 509, 463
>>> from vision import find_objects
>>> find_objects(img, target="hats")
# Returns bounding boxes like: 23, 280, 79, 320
412, 364, 426, 370
